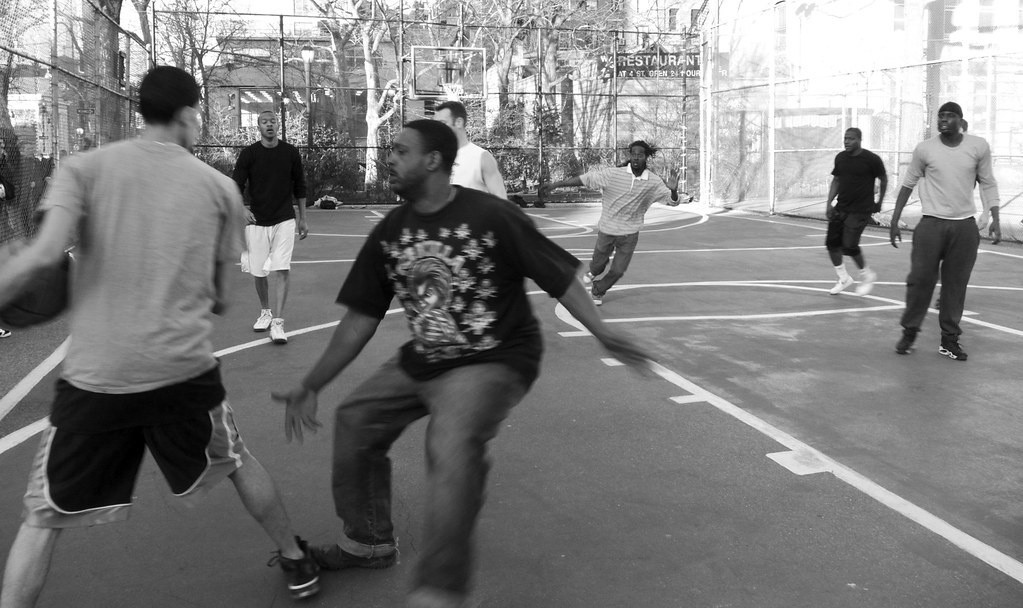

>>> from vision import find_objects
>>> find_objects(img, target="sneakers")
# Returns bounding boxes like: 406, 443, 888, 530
266, 535, 321, 600
895, 328, 921, 355
270, 318, 288, 344
582, 271, 595, 283
937, 337, 968, 361
830, 276, 854, 295
855, 272, 877, 296
311, 542, 397, 571
405, 570, 474, 608
592, 293, 603, 306
253, 309, 273, 332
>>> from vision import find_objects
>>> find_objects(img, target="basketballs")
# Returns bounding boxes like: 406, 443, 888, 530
0, 241, 73, 331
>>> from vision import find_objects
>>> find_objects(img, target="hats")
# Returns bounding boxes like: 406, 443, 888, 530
939, 102, 963, 119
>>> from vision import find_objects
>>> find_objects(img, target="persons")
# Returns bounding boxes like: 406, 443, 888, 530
271, 119, 657, 608
0, 68, 321, 608
825, 127, 888, 299
432, 101, 510, 201
230, 111, 310, 344
539, 140, 680, 307
0, 174, 13, 338
888, 102, 1003, 361
